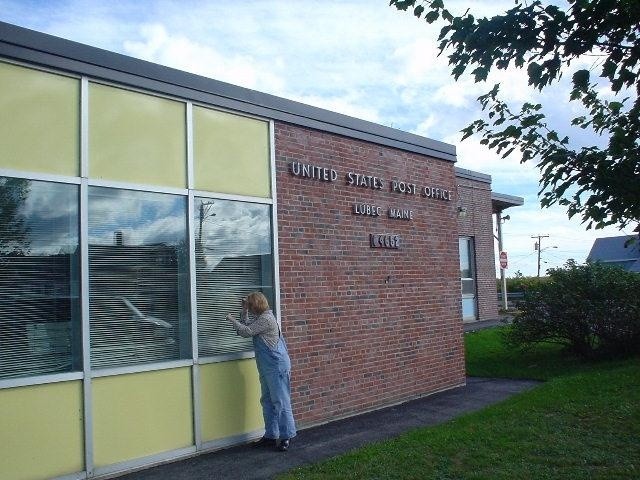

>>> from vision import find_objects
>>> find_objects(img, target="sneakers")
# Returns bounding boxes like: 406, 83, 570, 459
250, 436, 277, 449
278, 438, 291, 451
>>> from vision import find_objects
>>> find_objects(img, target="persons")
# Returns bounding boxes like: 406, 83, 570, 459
226, 292, 297, 452
